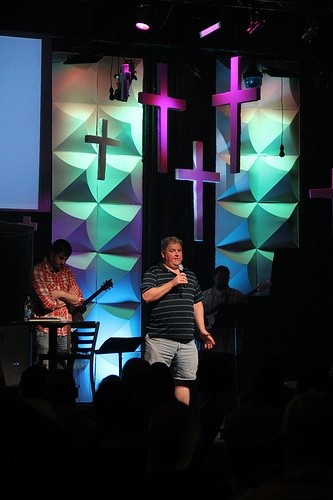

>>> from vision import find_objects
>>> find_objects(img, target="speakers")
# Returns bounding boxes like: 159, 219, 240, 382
0, 221, 37, 325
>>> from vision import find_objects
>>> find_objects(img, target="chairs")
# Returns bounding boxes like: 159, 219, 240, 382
37, 321, 100, 395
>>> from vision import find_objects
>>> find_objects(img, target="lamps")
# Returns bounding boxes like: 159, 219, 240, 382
244, 9, 266, 37
297, 15, 319, 48
109, 55, 137, 102
134, 0, 153, 31
243, 65, 263, 88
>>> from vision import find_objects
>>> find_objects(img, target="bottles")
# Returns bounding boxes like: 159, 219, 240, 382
24, 296, 32, 319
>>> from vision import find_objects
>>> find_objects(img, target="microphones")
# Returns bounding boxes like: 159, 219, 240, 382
178, 263, 187, 288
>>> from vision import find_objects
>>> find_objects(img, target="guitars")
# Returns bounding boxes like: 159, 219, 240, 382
71, 278, 114, 331
194, 279, 274, 344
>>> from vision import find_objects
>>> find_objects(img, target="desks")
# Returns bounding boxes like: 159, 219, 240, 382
33, 319, 81, 368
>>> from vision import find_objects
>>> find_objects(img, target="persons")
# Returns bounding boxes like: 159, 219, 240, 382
197, 265, 244, 352
140, 237, 215, 406
0, 355, 333, 499
32, 238, 84, 373
